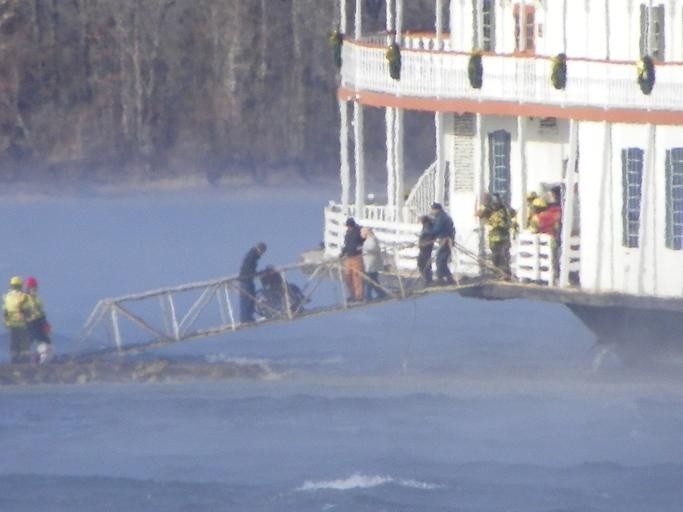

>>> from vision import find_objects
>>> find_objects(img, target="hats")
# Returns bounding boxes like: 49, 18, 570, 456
257, 242, 267, 253
343, 216, 355, 228
430, 202, 442, 210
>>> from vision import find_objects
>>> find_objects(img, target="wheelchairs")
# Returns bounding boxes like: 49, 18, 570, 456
252, 272, 301, 316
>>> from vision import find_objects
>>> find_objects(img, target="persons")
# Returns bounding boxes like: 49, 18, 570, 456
416, 213, 435, 287
338, 218, 364, 302
358, 226, 386, 300
24, 276, 54, 367
429, 202, 456, 285
238, 241, 267, 323
2, 275, 36, 363
475, 193, 518, 282
260, 266, 312, 305
523, 184, 561, 277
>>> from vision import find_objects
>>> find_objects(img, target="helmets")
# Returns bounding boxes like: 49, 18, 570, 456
526, 191, 537, 200
25, 277, 37, 288
8, 276, 23, 286
531, 197, 546, 208
